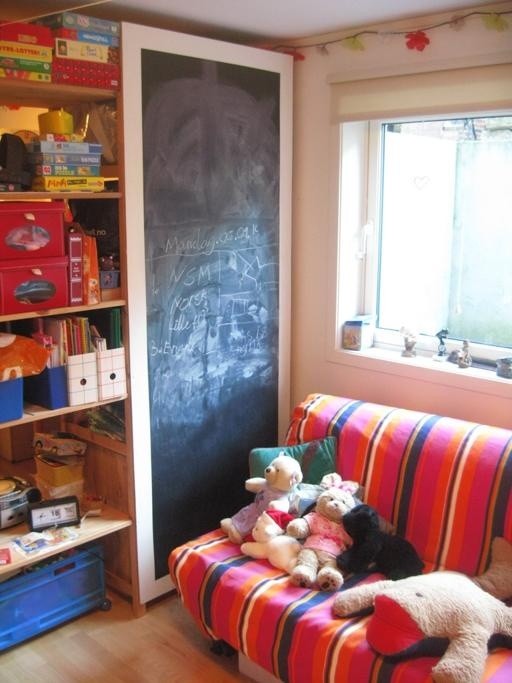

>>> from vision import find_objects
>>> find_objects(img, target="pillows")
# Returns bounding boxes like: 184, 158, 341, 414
248, 435, 337, 485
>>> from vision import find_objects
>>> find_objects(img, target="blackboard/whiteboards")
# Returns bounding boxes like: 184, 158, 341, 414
117, 21, 295, 606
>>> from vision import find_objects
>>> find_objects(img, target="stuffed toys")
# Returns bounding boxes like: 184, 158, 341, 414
241, 507, 302, 571
286, 472, 359, 591
329, 537, 512, 683
336, 503, 425, 580
221, 450, 303, 542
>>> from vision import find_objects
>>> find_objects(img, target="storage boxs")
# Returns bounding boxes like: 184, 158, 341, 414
0, 201, 67, 259
1, 541, 112, 653
1, 255, 70, 315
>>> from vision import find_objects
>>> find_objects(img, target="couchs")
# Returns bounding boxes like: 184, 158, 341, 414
168, 390, 512, 682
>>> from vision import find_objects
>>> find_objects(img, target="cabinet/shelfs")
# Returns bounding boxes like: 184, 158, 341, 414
0, 20, 293, 619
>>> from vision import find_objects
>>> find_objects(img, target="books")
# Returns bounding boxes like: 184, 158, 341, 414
1, 18, 54, 81
66, 230, 102, 306
33, 308, 119, 368
26, 138, 105, 195
30, 13, 122, 90
